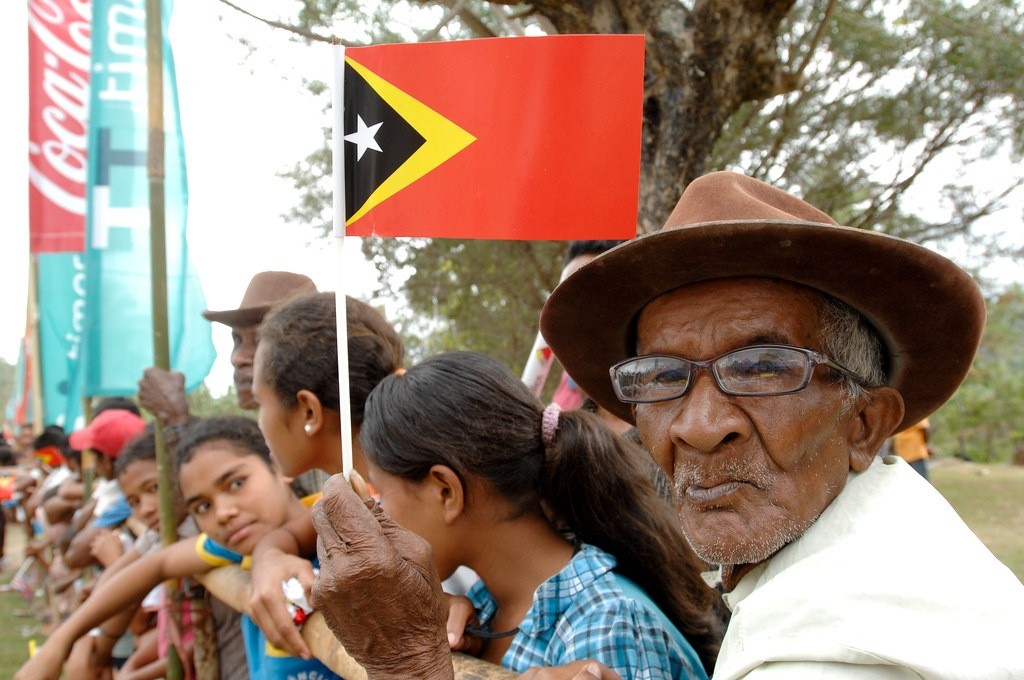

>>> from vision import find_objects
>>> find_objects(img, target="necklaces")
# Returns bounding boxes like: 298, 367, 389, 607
461, 539, 581, 638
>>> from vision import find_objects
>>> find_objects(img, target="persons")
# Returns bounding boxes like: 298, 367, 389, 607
0, 269, 732, 680
312, 167, 1024, 679
887, 417, 933, 483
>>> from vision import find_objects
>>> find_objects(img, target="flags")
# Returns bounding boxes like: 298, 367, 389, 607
344, 33, 646, 240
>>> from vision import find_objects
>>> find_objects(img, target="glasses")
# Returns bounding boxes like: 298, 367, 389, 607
610, 344, 880, 404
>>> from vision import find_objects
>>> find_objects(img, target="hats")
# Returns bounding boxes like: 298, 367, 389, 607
69, 411, 148, 458
540, 170, 987, 436
202, 271, 317, 326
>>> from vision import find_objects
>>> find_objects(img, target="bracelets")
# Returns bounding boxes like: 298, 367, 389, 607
90, 628, 125, 639
